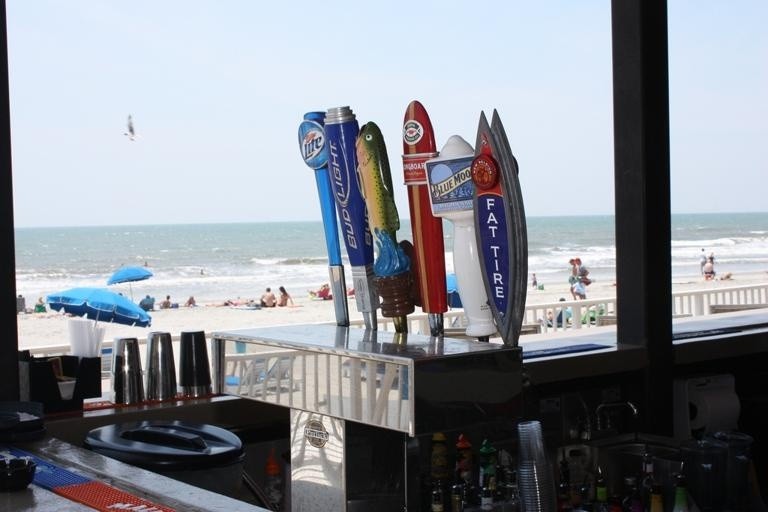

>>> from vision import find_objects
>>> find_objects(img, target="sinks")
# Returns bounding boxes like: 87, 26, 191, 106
606, 436, 704, 464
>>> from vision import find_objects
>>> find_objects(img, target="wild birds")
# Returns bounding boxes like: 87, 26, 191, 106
122, 114, 138, 142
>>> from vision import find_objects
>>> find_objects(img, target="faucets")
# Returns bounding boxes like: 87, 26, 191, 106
594, 398, 642, 432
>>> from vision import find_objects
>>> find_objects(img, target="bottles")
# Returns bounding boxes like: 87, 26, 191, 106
555, 449, 696, 512
431, 430, 522, 512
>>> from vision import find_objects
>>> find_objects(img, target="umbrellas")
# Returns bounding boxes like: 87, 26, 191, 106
46, 286, 153, 329
106, 265, 155, 302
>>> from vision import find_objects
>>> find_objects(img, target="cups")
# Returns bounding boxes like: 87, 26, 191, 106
179, 328, 212, 398
113, 336, 145, 405
518, 419, 554, 512
688, 430, 755, 511
146, 332, 177, 402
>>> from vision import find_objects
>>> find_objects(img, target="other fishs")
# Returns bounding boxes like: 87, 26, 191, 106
353, 120, 402, 247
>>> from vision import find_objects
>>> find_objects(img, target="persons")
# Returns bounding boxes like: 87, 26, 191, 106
155, 295, 173, 309
36, 296, 45, 312
716, 271, 733, 281
709, 252, 714, 265
276, 286, 294, 306
145, 295, 150, 299
699, 248, 706, 276
547, 297, 566, 322
703, 259, 716, 279
185, 295, 197, 308
259, 287, 278, 308
575, 257, 590, 299
17, 294, 27, 314
569, 258, 578, 301
531, 273, 538, 289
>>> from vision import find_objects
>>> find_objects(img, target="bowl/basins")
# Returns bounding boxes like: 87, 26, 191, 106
0, 458, 38, 493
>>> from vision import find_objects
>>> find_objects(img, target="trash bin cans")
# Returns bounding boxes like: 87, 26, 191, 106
84, 419, 247, 501
